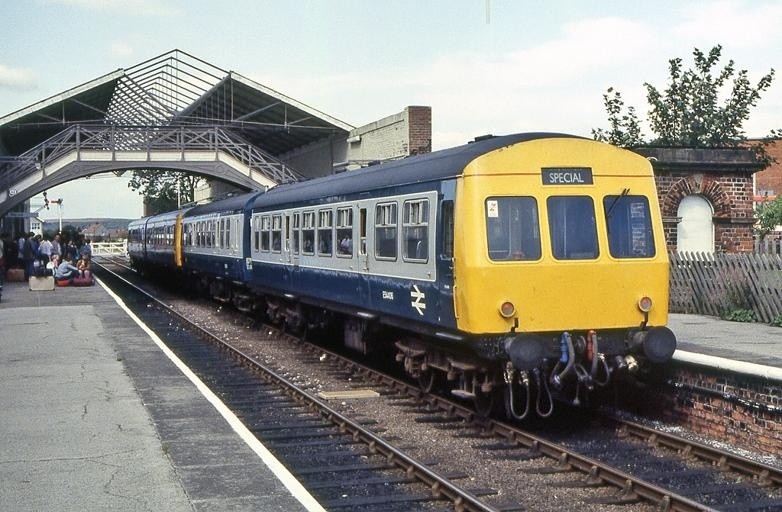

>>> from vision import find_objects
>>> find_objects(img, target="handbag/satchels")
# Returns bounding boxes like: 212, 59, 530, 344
33, 268, 53, 276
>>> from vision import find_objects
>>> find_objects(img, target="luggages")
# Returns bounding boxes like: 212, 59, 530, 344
7, 268, 24, 281
29, 276, 55, 291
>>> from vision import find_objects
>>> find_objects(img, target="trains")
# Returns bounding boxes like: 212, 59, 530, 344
127, 131, 677, 421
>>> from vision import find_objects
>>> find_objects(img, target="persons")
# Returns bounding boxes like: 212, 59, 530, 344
263, 231, 353, 255
0, 229, 92, 288
